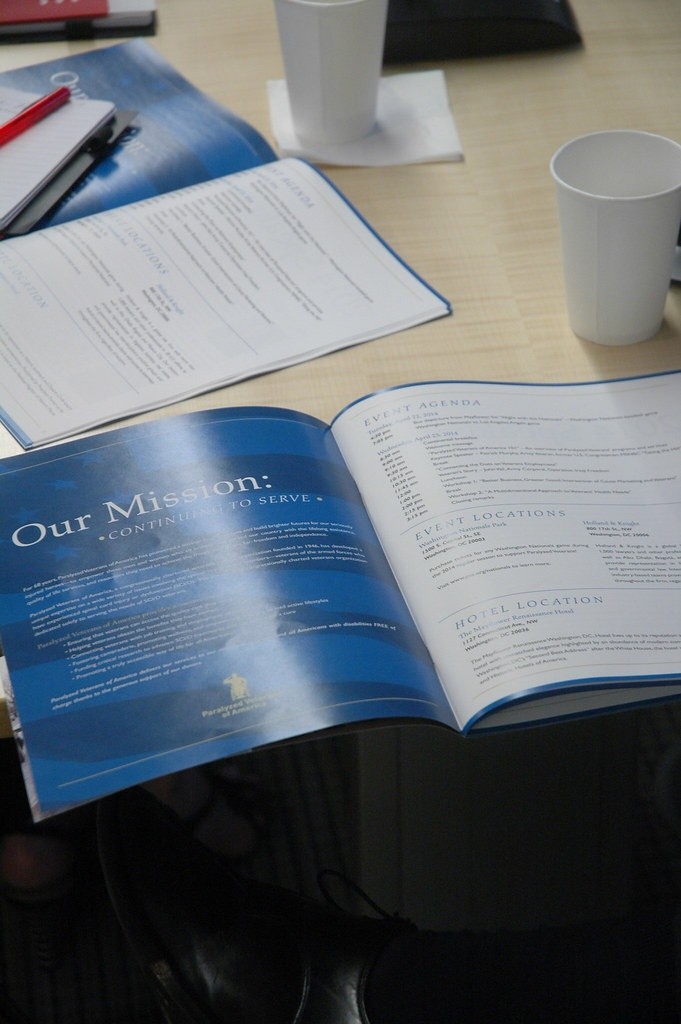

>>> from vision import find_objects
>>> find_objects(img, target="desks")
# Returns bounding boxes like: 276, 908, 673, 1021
0, 0, 680, 738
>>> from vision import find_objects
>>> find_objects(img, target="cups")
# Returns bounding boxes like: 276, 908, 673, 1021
268, 0, 389, 142
547, 128, 681, 348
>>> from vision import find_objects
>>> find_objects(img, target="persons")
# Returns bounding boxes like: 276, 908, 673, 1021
0, 731, 284, 911
97, 784, 681, 1024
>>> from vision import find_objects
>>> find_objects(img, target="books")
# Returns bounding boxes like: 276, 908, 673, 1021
0, 0, 157, 45
0, 88, 140, 235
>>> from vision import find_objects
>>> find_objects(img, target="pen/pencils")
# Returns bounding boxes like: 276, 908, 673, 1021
0, 86, 71, 145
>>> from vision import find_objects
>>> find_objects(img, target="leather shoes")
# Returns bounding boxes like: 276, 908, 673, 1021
96, 786, 421, 1024
